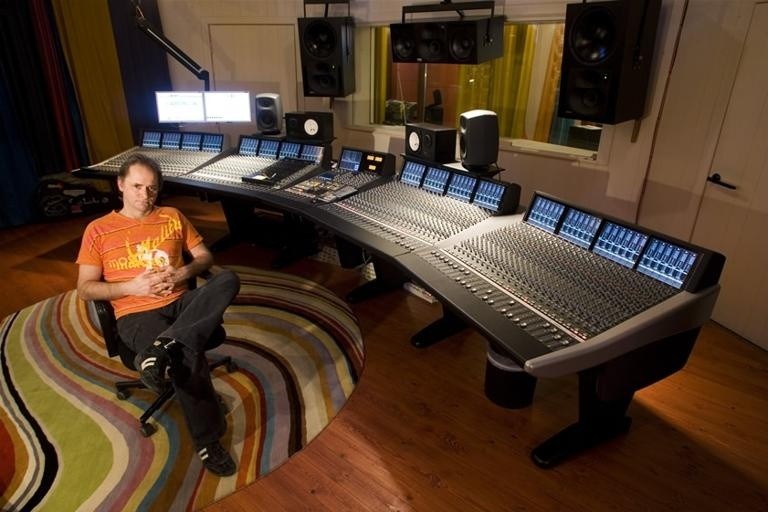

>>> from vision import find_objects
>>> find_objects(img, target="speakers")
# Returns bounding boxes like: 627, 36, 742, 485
285, 111, 333, 144
566, 124, 602, 152
459, 109, 499, 171
298, 16, 355, 97
405, 123, 457, 164
390, 15, 504, 64
425, 106, 443, 125
255, 93, 284, 138
557, 0, 662, 126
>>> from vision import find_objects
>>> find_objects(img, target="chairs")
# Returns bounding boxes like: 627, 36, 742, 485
81, 251, 238, 438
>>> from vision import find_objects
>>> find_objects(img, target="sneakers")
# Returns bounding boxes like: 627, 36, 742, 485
133, 346, 168, 396
194, 441, 236, 476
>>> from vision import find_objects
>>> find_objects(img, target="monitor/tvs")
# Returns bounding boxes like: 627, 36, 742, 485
153, 90, 206, 125
203, 90, 253, 125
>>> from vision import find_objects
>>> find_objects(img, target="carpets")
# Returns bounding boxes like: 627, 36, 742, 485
0, 260, 364, 512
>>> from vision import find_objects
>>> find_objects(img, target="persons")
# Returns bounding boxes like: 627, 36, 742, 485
75, 154, 242, 477
